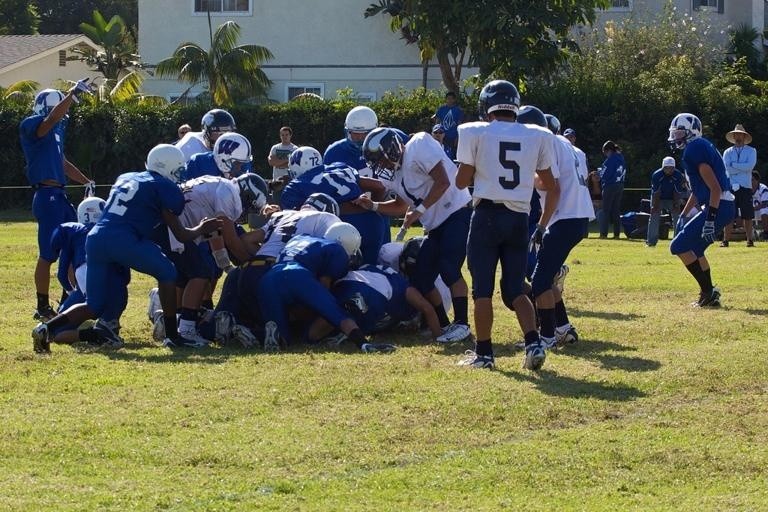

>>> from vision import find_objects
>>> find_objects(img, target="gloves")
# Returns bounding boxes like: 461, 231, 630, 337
213, 248, 236, 273
701, 219, 715, 243
83, 179, 97, 197
530, 227, 545, 259
675, 215, 685, 233
395, 225, 407, 241
69, 76, 93, 106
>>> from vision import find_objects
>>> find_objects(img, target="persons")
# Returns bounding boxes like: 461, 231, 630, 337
32, 144, 225, 353
20, 78, 96, 323
150, 92, 471, 355
50, 196, 125, 350
668, 112, 736, 309
457, 79, 603, 371
599, 140, 626, 240
646, 125, 768, 247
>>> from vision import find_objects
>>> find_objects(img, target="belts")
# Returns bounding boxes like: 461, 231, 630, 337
243, 260, 266, 268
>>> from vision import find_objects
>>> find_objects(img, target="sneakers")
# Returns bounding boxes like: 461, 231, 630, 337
693, 289, 720, 308
147, 288, 287, 353
710, 301, 720, 308
32, 301, 126, 353
720, 241, 728, 247
398, 314, 471, 342
318, 294, 396, 353
456, 350, 493, 371
746, 241, 753, 247
517, 324, 579, 370
554, 264, 568, 296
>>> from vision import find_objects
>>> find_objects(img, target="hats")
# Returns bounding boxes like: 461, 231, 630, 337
563, 128, 575, 138
725, 125, 752, 145
662, 157, 676, 170
432, 124, 445, 133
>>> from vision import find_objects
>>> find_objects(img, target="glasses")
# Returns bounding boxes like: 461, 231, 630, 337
434, 132, 442, 134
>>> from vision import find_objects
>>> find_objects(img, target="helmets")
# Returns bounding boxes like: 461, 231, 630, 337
344, 105, 380, 142
145, 106, 269, 215
33, 89, 65, 117
288, 146, 362, 254
474, 81, 561, 134
75, 198, 104, 224
362, 127, 403, 169
667, 112, 704, 155
378, 243, 404, 272
